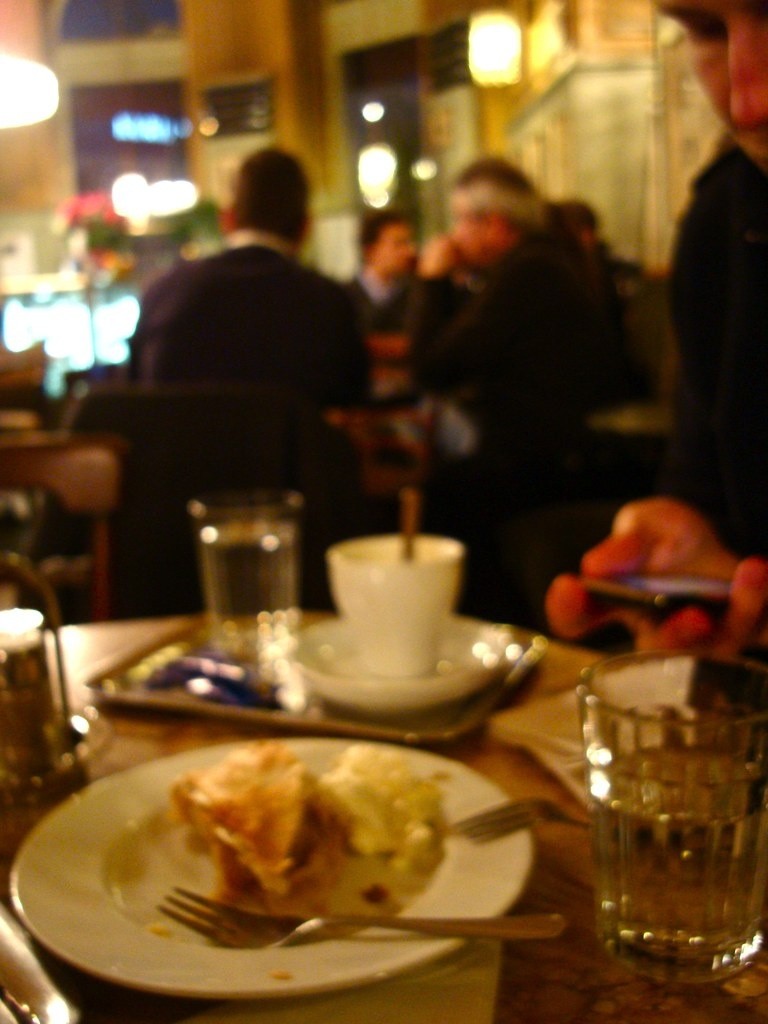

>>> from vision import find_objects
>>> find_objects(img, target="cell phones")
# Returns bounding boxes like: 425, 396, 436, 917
583, 570, 733, 612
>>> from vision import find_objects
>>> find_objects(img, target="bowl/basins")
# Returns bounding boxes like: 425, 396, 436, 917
282, 619, 516, 717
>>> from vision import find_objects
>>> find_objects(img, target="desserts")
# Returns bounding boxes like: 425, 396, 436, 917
181, 734, 448, 931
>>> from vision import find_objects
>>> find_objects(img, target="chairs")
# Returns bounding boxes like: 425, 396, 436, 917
0, 436, 117, 625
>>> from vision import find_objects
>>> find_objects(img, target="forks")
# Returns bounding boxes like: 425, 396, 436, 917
452, 799, 590, 846
157, 887, 565, 942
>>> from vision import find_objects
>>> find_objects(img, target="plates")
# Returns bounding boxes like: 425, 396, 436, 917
9, 739, 535, 999
492, 687, 584, 805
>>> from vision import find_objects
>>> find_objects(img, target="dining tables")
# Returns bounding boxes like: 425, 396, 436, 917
1, 611, 766, 1022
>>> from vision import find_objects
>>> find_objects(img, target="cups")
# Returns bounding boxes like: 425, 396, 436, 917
578, 648, 768, 983
188, 491, 300, 657
0, 608, 57, 769
327, 532, 464, 675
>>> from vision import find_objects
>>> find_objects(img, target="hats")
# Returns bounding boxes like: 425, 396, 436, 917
450, 156, 541, 229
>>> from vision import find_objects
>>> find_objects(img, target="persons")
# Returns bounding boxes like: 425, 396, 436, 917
121, 138, 376, 608
542, 0, 768, 661
345, 153, 656, 605
